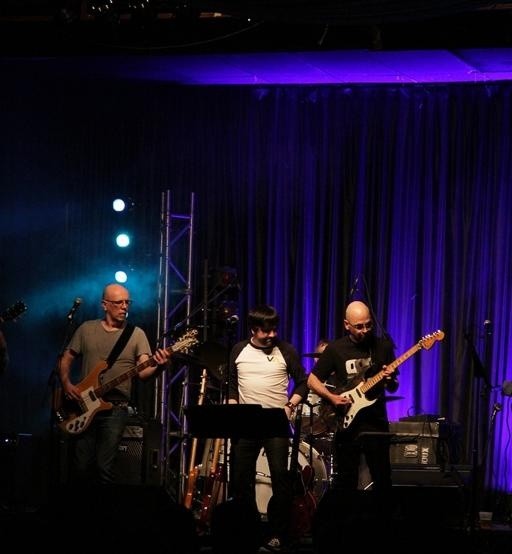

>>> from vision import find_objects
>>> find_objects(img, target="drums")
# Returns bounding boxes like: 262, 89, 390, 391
256, 439, 330, 520
289, 389, 338, 436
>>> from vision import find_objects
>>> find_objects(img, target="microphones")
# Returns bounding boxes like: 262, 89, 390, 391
65, 296, 83, 320
227, 314, 239, 323
346, 279, 358, 302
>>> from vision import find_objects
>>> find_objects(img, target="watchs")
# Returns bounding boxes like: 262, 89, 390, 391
285, 402, 297, 414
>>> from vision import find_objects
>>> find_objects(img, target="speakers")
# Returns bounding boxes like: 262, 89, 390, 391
115, 416, 163, 489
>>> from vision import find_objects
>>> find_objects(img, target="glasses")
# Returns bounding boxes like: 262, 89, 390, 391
104, 300, 132, 306
348, 320, 373, 330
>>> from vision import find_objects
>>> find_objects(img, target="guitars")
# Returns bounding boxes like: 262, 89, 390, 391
184, 369, 225, 533
53, 329, 199, 434
319, 330, 445, 428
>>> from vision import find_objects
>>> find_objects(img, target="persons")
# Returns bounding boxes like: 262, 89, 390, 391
0, 481, 473, 553
225, 302, 309, 521
300, 338, 337, 418
53, 280, 173, 497
304, 298, 400, 504
